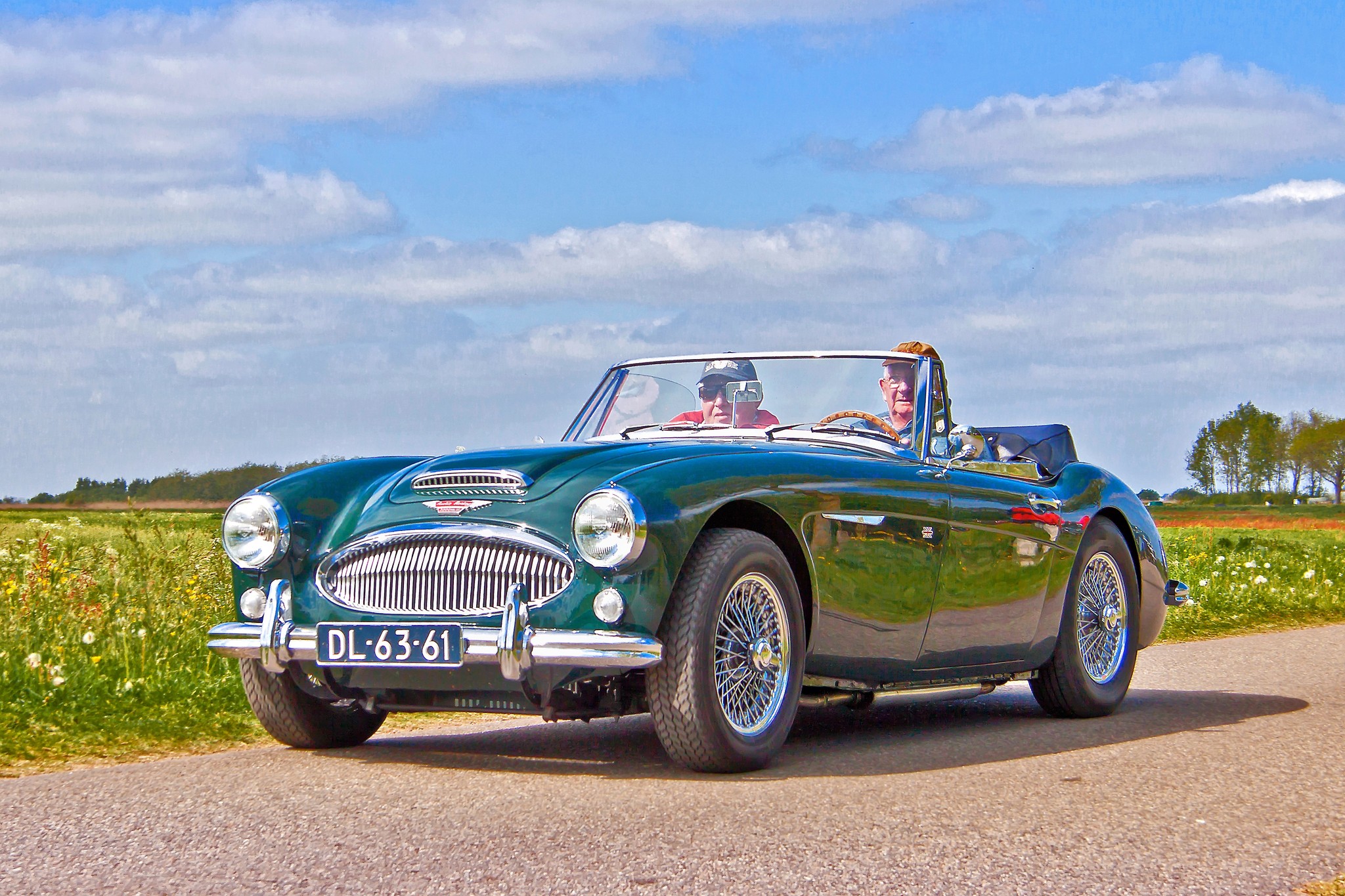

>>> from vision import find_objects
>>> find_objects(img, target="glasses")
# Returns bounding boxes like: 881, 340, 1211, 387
885, 374, 917, 387
699, 385, 727, 399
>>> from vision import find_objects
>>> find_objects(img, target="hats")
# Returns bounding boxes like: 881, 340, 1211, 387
695, 351, 759, 382
881, 343, 942, 364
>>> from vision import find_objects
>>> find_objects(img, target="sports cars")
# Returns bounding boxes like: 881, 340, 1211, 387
214, 344, 1190, 762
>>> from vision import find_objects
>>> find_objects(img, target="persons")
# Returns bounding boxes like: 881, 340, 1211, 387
652, 347, 780, 433
841, 341, 994, 461
